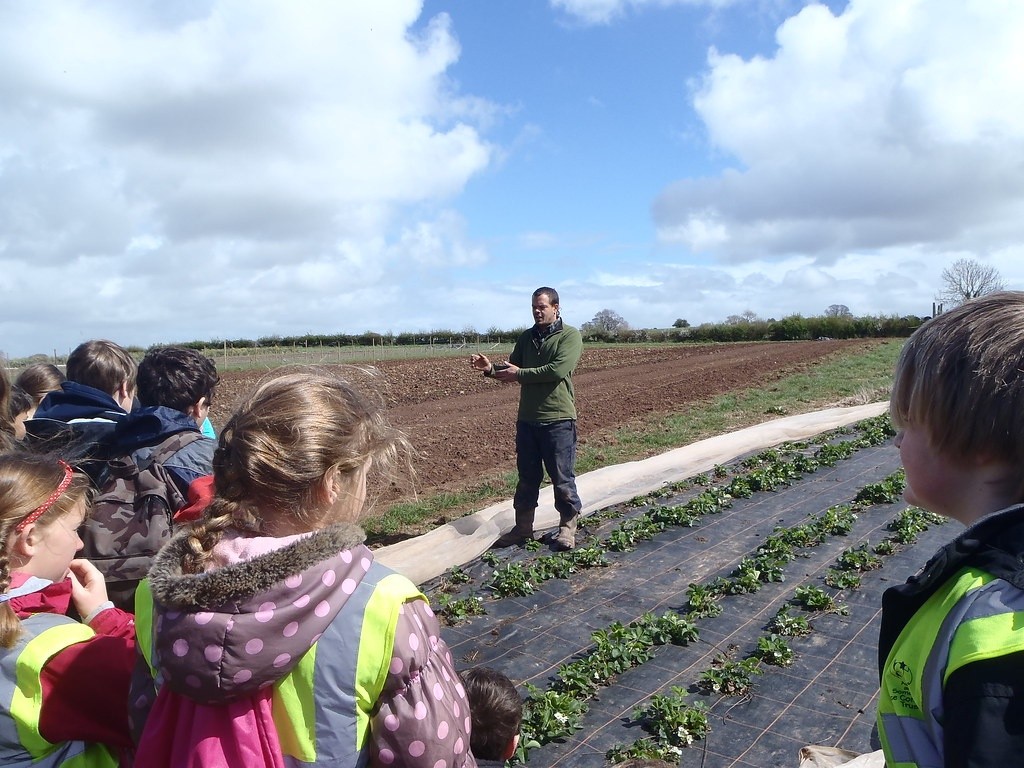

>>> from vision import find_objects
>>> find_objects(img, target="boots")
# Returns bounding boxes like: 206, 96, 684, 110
496, 509, 535, 548
553, 511, 579, 550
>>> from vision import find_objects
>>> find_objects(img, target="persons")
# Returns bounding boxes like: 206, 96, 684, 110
875, 290, 1024, 768
127, 373, 477, 768
0, 451, 136, 768
0, 340, 219, 627
457, 668, 522, 768
470, 286, 582, 550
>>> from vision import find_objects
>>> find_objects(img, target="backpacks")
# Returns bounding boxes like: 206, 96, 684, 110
73, 431, 206, 615
